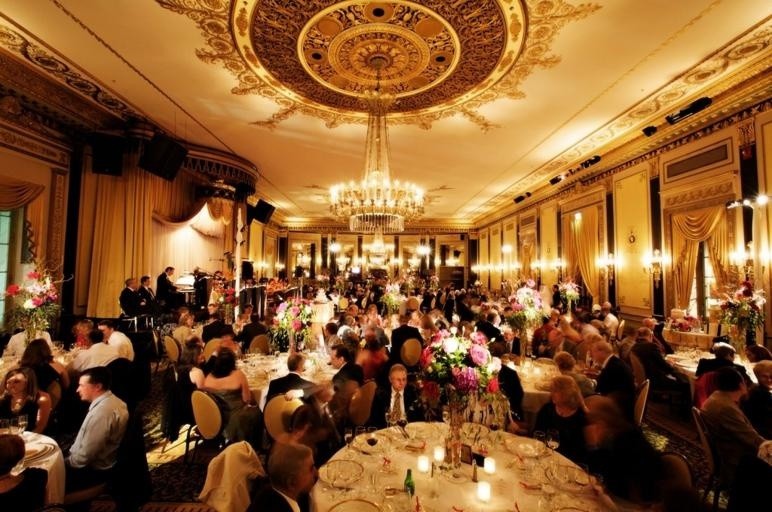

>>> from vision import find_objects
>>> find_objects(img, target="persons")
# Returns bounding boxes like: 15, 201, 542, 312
2, 265, 771, 511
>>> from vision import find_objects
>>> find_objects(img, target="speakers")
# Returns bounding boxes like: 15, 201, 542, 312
294, 266, 304, 276
242, 261, 254, 279
254, 199, 275, 225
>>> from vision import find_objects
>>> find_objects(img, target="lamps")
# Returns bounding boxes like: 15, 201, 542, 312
638, 249, 672, 288
327, 59, 424, 248
593, 253, 621, 286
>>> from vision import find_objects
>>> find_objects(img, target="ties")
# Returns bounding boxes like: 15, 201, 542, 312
390, 391, 405, 423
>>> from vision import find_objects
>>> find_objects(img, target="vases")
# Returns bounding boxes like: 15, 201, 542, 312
731, 323, 749, 359
20, 315, 37, 347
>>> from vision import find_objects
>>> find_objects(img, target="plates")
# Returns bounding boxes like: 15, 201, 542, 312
318, 418, 598, 512
22, 440, 55, 465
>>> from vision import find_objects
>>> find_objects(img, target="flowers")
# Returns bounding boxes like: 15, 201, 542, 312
720, 281, 767, 345
3, 263, 64, 344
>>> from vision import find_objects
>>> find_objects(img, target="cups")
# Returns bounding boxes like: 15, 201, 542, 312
1, 413, 31, 440
